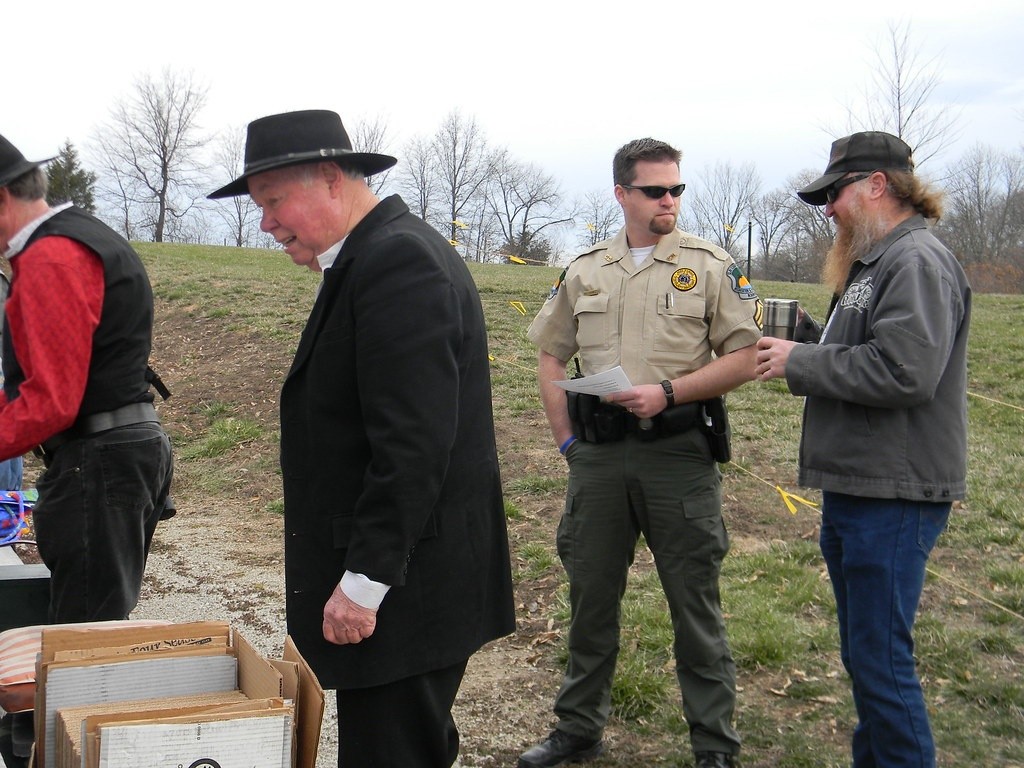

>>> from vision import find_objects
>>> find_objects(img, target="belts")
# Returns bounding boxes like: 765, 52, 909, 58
33, 403, 160, 458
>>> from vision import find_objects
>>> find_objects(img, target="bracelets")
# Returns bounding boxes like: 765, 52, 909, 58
660, 380, 675, 406
560, 435, 576, 455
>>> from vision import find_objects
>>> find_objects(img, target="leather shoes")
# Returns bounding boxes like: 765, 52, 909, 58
695, 749, 733, 768
518, 730, 603, 768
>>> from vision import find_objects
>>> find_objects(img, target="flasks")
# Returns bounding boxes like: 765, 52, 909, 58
762, 298, 799, 362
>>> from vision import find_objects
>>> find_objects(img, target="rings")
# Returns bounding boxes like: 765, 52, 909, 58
629, 407, 632, 412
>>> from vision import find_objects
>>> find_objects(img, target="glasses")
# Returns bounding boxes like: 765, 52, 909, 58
622, 183, 685, 199
823, 173, 869, 204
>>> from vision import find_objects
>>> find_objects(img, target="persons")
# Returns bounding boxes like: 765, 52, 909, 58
524, 137, 763, 768
205, 110, 517, 768
755, 130, 972, 768
0, 134, 178, 626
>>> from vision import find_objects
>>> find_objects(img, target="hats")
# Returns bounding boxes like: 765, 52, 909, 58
206, 110, 397, 199
0, 135, 62, 182
797, 131, 913, 206
